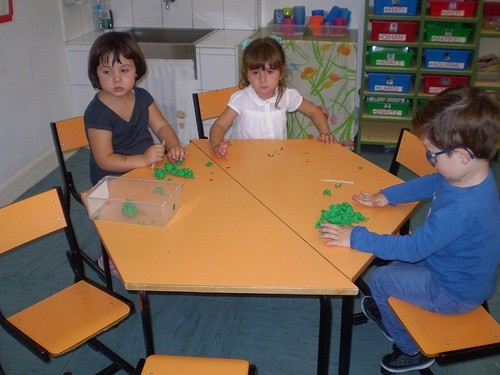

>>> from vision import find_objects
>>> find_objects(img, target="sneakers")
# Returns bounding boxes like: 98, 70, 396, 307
361, 296, 394, 342
381, 348, 436, 374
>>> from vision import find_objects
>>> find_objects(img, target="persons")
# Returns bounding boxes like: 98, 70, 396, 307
316, 81, 500, 374
209, 35, 337, 159
83, 31, 189, 282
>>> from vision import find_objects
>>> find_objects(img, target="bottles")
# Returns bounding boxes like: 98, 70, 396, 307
95, 0, 114, 33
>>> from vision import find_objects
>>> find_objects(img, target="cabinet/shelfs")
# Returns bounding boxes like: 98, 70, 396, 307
357, 0, 483, 154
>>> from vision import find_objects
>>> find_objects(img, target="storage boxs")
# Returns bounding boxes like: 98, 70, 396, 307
366, 0, 478, 118
87, 175, 182, 228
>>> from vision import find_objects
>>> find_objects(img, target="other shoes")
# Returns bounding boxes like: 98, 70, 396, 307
98, 253, 120, 278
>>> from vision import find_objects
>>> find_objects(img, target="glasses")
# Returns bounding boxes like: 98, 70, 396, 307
426, 146, 474, 167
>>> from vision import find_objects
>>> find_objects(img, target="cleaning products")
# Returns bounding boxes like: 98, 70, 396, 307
92, 0, 105, 31
103, 0, 113, 29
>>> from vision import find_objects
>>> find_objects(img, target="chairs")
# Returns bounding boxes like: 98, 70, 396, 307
0, 85, 500, 375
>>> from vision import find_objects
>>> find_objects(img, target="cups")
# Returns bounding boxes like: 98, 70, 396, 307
273, 6, 352, 35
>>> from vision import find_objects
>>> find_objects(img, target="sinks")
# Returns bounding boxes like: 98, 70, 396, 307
125, 27, 218, 79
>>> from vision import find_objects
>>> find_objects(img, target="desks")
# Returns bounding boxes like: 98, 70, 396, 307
81, 139, 360, 375
189, 139, 421, 374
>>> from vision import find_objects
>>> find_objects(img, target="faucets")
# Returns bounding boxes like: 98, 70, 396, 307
162, 0, 175, 9
